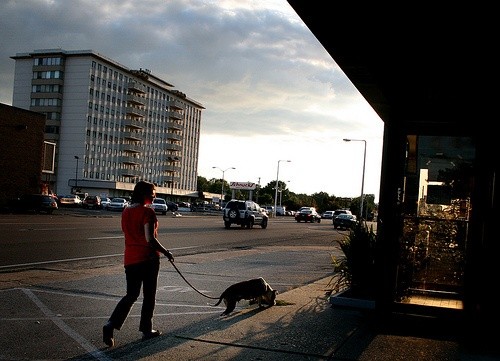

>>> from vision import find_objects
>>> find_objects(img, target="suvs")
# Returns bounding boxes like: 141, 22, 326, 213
223, 199, 268, 229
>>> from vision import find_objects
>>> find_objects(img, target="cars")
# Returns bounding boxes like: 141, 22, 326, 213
108, 198, 128, 210
295, 206, 321, 223
333, 210, 357, 229
152, 198, 178, 215
83, 195, 111, 208
60, 194, 82, 206
323, 211, 335, 218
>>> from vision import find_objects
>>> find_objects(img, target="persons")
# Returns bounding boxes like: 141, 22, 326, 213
102, 181, 175, 347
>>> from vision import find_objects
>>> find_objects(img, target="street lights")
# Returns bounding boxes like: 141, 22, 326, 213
343, 138, 367, 223
274, 159, 292, 216
280, 180, 291, 207
74, 155, 79, 189
212, 166, 236, 207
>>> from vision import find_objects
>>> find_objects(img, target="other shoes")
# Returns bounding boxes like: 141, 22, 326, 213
103, 325, 114, 347
145, 331, 162, 337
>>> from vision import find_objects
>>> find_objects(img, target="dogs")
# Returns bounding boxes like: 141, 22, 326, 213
208, 277, 278, 316
